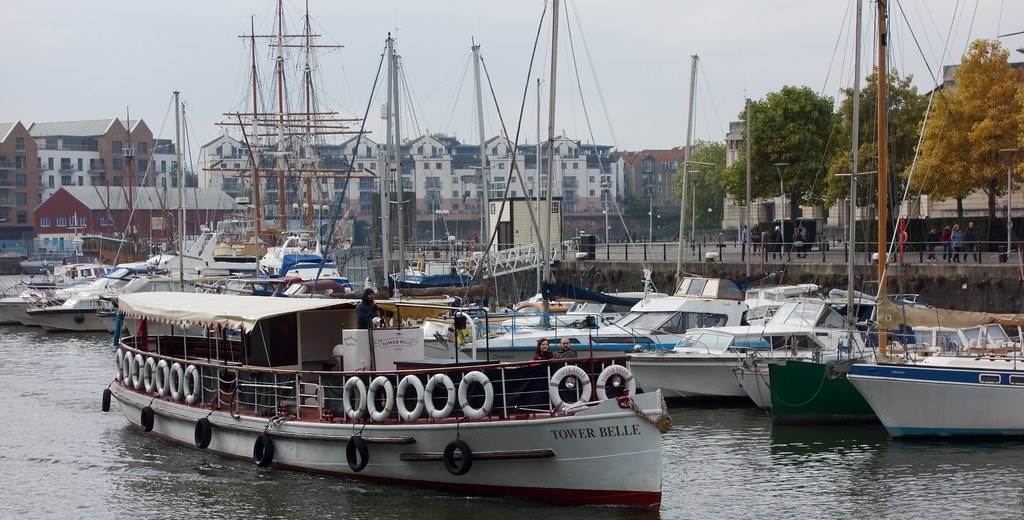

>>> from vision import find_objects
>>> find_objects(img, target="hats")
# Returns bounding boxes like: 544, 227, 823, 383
366, 289, 374, 296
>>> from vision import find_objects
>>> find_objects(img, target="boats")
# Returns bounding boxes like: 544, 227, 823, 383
106, 292, 663, 510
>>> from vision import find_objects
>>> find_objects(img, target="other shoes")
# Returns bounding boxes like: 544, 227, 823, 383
964, 258, 967, 263
927, 258, 931, 260
973, 260, 978, 263
957, 260, 961, 263
932, 258, 937, 261
943, 259, 947, 261
952, 258, 956, 263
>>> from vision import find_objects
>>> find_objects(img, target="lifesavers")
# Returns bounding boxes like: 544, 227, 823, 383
549, 364, 636, 412
442, 440, 473, 475
343, 371, 493, 418
346, 436, 369, 472
252, 433, 275, 466
102, 389, 112, 410
114, 349, 200, 404
141, 406, 154, 432
194, 418, 212, 448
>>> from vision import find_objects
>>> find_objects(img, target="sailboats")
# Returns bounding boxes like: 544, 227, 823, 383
0, 0, 1024, 443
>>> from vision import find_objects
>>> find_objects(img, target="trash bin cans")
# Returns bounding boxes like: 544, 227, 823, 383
999, 246, 1007, 262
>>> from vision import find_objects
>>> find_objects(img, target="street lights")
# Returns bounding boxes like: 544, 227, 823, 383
1000, 148, 1018, 258
601, 181, 611, 243
773, 163, 791, 256
708, 208, 712, 242
687, 170, 703, 255
645, 184, 654, 250
603, 209, 606, 244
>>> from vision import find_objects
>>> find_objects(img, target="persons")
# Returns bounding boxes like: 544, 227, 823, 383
942, 224, 962, 262
356, 289, 379, 329
793, 225, 809, 258
555, 338, 576, 359
529, 339, 555, 364
925, 228, 939, 262
770, 226, 783, 259
963, 222, 977, 262
742, 225, 761, 256
